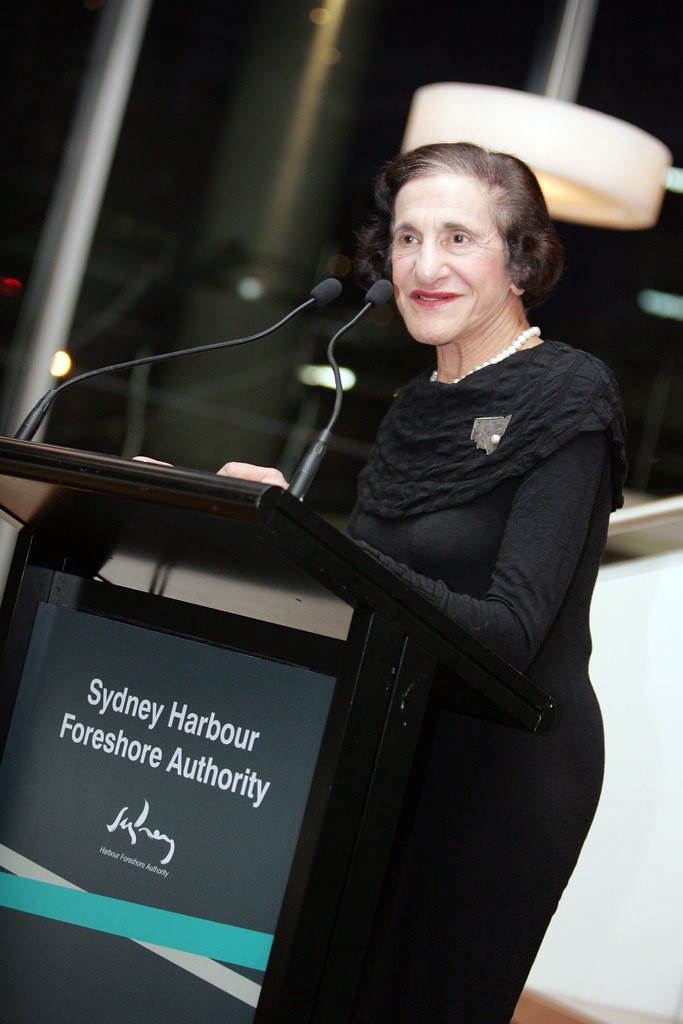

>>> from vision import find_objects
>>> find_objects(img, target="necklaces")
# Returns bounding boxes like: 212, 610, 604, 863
430, 326, 541, 384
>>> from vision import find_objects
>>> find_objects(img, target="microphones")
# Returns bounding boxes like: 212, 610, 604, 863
287, 281, 393, 501
14, 277, 343, 442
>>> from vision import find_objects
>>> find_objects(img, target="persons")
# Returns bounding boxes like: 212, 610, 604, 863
132, 141, 631, 1024
